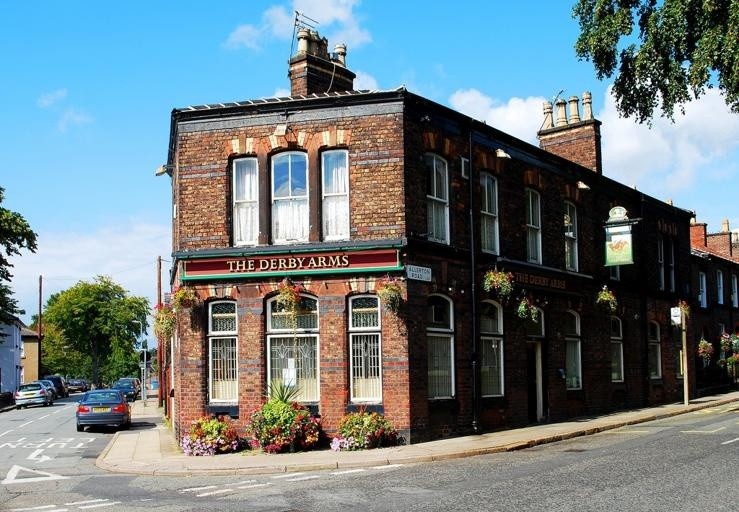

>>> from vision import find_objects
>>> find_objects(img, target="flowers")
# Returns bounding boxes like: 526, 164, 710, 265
273, 278, 310, 353
378, 278, 407, 318
181, 413, 247, 457
696, 331, 738, 383
483, 268, 540, 325
597, 283, 619, 312
251, 395, 321, 451
328, 399, 401, 451
676, 300, 693, 321
152, 285, 200, 341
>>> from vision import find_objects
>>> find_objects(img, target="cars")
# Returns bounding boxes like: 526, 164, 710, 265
15, 375, 141, 431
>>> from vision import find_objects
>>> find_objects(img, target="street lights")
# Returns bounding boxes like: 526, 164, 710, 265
132, 320, 148, 390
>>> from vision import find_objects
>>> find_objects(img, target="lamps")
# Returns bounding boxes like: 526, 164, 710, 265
155, 165, 174, 176
275, 123, 297, 144
